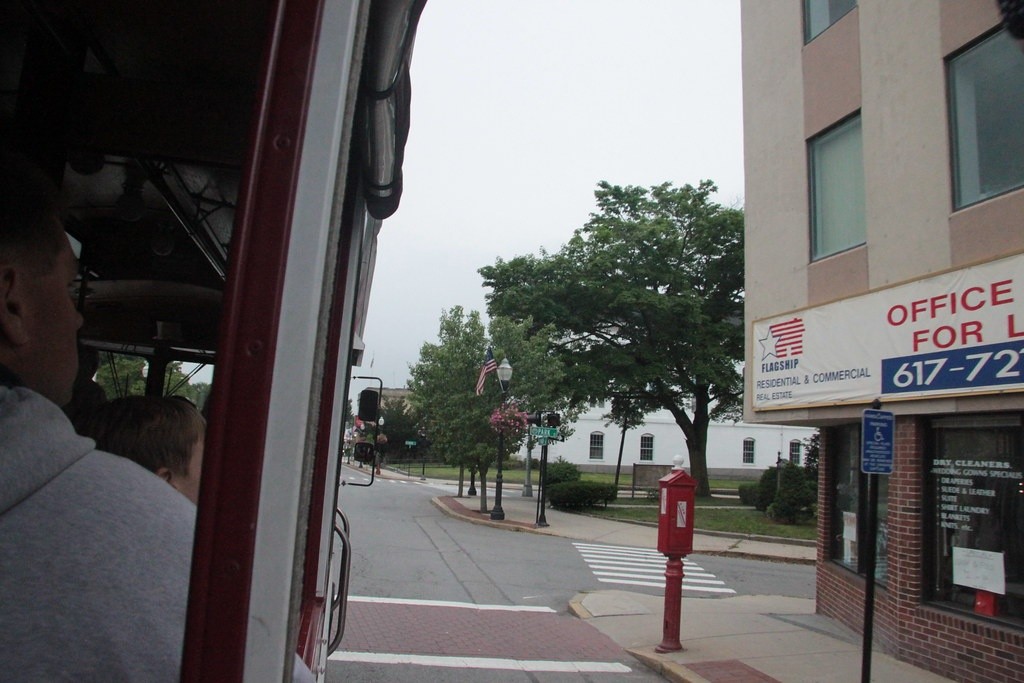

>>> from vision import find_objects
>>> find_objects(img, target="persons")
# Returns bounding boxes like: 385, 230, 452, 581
0, 144, 317, 682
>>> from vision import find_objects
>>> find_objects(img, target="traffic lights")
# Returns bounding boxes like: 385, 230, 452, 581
548, 413, 560, 427
526, 413, 541, 427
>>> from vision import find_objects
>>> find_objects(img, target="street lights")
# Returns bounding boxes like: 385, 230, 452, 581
375, 417, 384, 452
490, 355, 513, 520
359, 424, 365, 468
420, 433, 426, 480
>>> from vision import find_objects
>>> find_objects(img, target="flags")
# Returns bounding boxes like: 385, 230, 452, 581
475, 348, 495, 396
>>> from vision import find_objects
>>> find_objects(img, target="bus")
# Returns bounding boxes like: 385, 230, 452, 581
0, 0, 425, 683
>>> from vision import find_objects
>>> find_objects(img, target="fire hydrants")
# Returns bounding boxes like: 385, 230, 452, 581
376, 462, 382, 475
